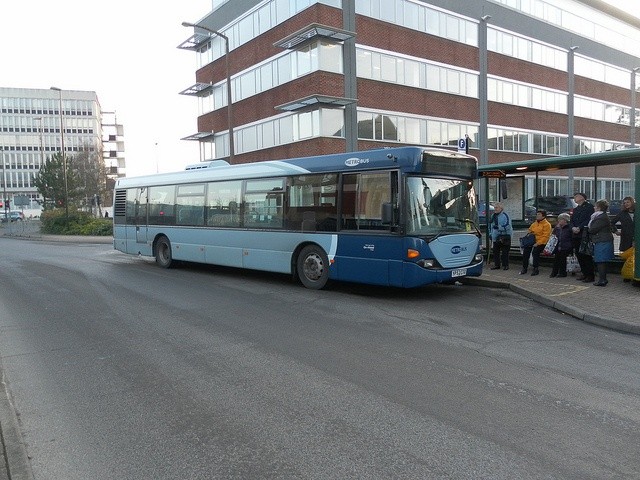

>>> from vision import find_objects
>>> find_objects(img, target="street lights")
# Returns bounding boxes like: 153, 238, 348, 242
50, 87, 70, 231
182, 22, 234, 165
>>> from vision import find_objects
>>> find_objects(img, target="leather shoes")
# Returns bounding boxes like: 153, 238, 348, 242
551, 271, 556, 277
583, 279, 591, 282
491, 266, 500, 270
576, 278, 584, 280
504, 265, 509, 270
531, 267, 538, 275
518, 264, 527, 274
594, 281, 606, 285
558, 272, 567, 277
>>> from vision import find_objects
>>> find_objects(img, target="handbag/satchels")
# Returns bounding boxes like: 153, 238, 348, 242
520, 233, 535, 247
566, 246, 581, 273
543, 234, 558, 256
619, 247, 640, 281
578, 227, 594, 256
496, 226, 511, 248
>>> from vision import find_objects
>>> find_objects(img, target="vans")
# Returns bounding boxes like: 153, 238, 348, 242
525, 197, 578, 218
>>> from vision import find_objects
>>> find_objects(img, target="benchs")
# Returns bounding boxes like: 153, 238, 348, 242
481, 244, 626, 265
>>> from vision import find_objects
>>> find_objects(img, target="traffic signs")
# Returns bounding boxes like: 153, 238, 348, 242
458, 138, 466, 152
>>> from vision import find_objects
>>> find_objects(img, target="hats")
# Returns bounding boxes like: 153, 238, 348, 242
574, 193, 586, 200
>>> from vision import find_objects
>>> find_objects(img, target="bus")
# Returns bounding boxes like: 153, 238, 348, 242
113, 147, 483, 289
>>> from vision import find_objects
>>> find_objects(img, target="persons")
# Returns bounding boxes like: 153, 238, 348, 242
611, 196, 636, 282
549, 213, 572, 278
519, 209, 552, 276
587, 199, 615, 286
569, 193, 595, 283
488, 201, 513, 270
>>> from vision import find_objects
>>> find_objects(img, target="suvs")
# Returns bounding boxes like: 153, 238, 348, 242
478, 201, 494, 224
0, 212, 23, 222
